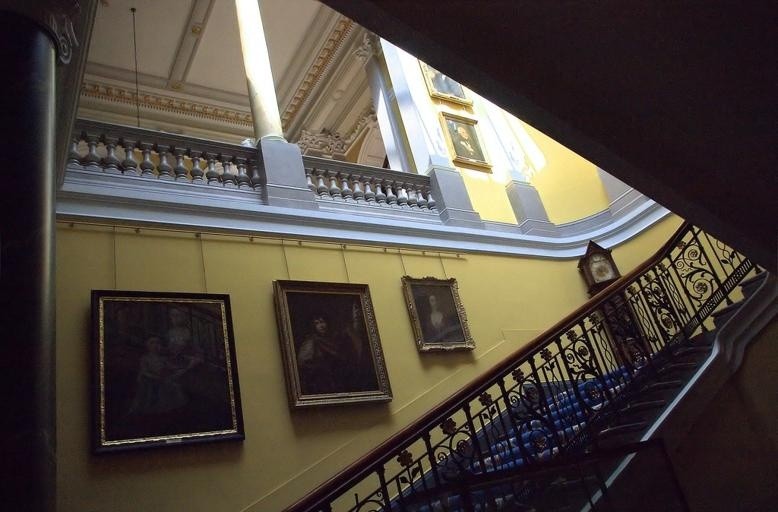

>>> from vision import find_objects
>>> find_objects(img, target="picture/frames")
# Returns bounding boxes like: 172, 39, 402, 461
418, 60, 473, 107
89, 289, 246, 452
438, 110, 493, 169
401, 276, 477, 353
273, 279, 393, 409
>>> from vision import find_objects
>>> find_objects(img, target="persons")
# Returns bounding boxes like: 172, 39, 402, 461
296, 307, 360, 397
423, 292, 457, 342
102, 302, 222, 418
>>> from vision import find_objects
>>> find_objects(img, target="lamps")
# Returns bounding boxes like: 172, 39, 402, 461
578, 238, 655, 366
